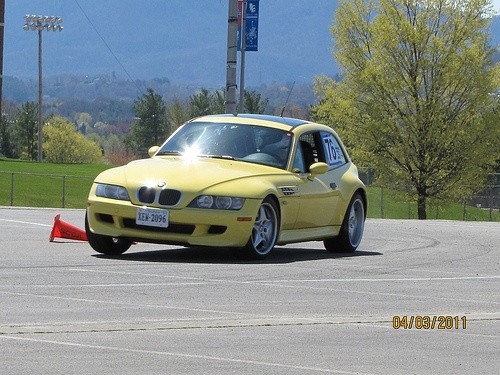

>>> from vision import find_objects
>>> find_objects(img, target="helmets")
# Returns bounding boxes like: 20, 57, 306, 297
259, 131, 290, 153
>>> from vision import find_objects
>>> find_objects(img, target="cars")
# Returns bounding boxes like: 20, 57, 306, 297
85, 107, 368, 259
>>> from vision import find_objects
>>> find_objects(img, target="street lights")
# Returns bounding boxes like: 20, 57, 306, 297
23, 13, 64, 163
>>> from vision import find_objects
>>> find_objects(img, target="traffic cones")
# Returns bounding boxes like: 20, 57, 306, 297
50, 213, 137, 245
